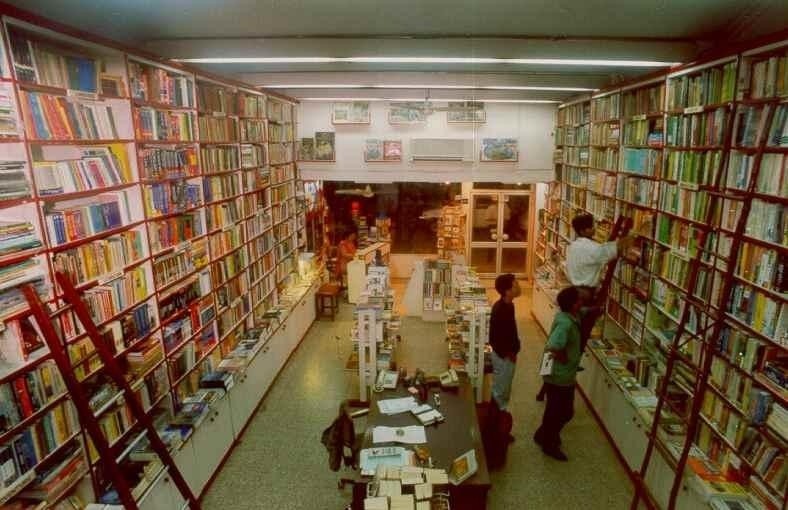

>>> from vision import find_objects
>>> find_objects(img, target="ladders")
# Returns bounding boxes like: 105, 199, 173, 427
536, 215, 634, 401
19, 269, 202, 510
629, 95, 780, 510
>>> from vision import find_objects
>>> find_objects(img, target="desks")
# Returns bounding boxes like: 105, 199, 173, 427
347, 264, 493, 510
346, 238, 394, 306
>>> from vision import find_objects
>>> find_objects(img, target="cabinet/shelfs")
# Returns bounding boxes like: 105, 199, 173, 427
435, 203, 468, 260
530, 43, 788, 508
2, 7, 334, 508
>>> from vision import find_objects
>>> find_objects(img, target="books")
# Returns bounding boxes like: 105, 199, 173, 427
351, 266, 401, 355
128, 60, 295, 255
535, 79, 662, 389
153, 202, 313, 418
420, 257, 496, 375
1, 230, 210, 505
658, 52, 788, 250
647, 215, 788, 399
2, 24, 139, 256
635, 338, 788, 509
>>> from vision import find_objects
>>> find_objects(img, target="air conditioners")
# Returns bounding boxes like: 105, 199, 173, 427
408, 138, 476, 165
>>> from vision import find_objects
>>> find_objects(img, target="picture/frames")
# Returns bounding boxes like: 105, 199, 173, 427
314, 132, 336, 163
480, 137, 520, 162
384, 138, 403, 162
362, 137, 384, 162
297, 137, 314, 161
443, 99, 489, 125
387, 101, 427, 124
330, 99, 372, 125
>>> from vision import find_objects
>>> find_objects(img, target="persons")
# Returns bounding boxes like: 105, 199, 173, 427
335, 231, 357, 279
487, 273, 524, 413
532, 211, 638, 461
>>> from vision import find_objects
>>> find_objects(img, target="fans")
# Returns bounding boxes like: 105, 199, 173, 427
388, 89, 480, 115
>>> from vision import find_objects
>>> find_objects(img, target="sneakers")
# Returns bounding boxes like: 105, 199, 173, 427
534, 432, 566, 461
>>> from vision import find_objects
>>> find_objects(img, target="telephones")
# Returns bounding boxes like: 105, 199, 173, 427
372, 368, 400, 392
438, 369, 459, 391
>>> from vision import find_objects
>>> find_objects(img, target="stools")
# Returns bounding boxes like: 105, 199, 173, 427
313, 281, 340, 323
